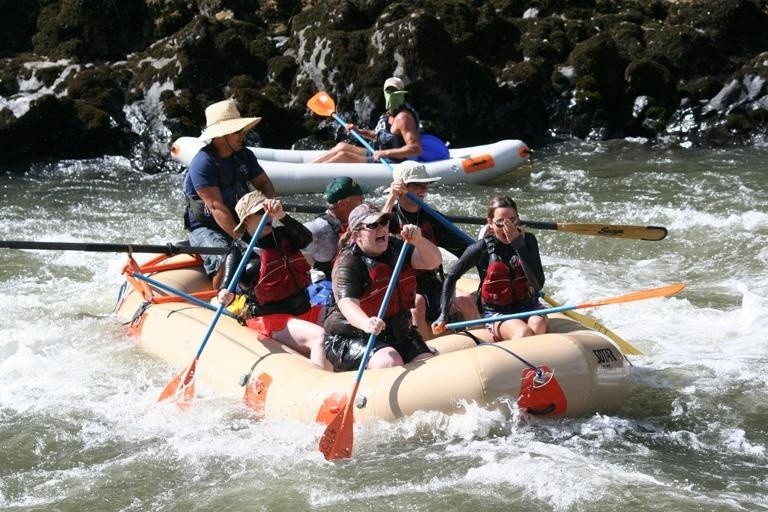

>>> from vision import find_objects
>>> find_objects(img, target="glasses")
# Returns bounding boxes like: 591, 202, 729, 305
492, 216, 519, 228
355, 219, 389, 229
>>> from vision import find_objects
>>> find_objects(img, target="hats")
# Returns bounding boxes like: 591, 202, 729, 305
348, 203, 392, 232
383, 77, 404, 90
233, 189, 281, 234
323, 175, 370, 204
198, 100, 262, 145
383, 160, 442, 193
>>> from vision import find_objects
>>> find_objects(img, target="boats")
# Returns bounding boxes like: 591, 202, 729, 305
171, 136, 531, 194
115, 239, 630, 443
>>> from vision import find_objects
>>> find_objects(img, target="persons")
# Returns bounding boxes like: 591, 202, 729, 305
323, 202, 442, 372
298, 176, 364, 310
216, 191, 333, 372
372, 159, 479, 340
313, 77, 422, 163
431, 196, 548, 341
184, 100, 275, 292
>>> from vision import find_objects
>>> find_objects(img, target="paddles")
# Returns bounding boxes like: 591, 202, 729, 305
433, 283, 685, 332
280, 203, 667, 242
400, 192, 642, 356
319, 238, 411, 460
157, 210, 268, 409
306, 90, 393, 174
